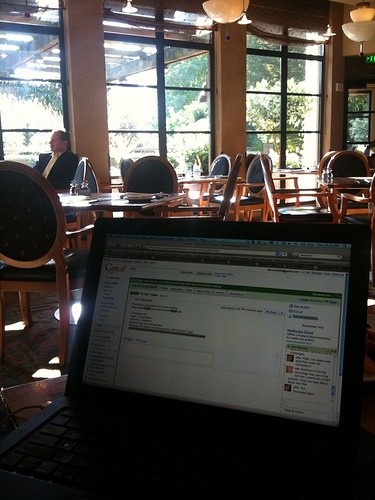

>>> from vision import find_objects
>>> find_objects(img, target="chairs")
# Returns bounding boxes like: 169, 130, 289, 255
0, 150, 375, 372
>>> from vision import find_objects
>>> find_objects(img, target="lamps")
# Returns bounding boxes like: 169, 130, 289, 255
122, 0, 138, 15
341, 0, 375, 55
202, 0, 252, 40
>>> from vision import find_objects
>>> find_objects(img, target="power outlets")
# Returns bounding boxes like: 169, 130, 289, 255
335, 83, 346, 92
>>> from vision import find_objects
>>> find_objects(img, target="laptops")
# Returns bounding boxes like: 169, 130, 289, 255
0, 219, 372, 500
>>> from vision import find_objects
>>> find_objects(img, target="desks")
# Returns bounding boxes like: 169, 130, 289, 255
274, 167, 319, 174
315, 176, 371, 223
57, 191, 187, 219
176, 175, 242, 207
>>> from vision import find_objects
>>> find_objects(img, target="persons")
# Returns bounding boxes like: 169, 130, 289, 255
31, 130, 79, 189
